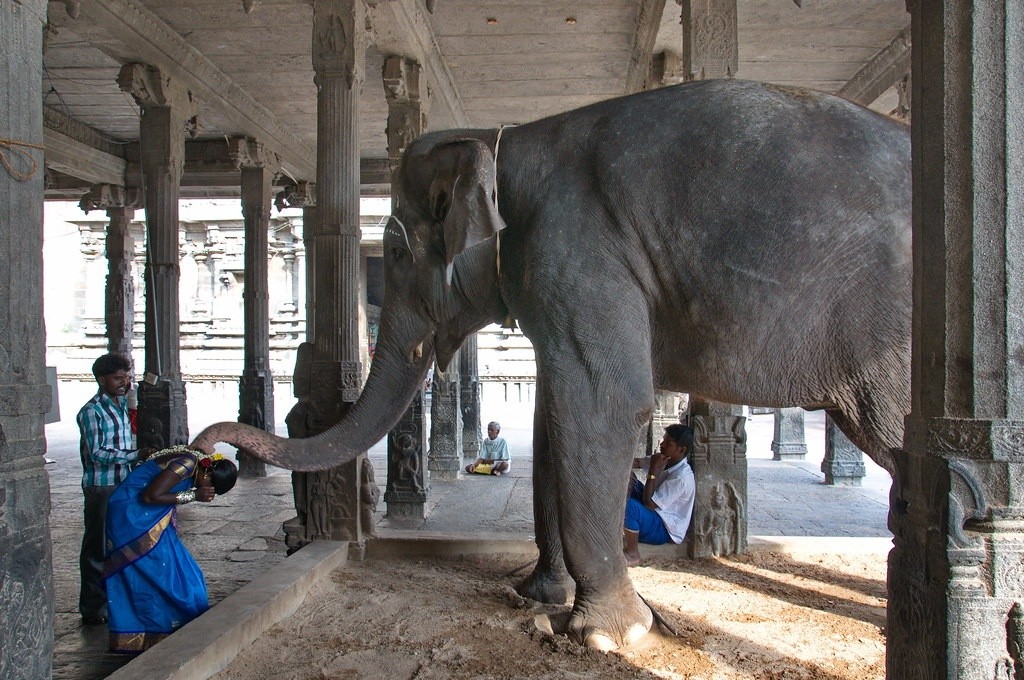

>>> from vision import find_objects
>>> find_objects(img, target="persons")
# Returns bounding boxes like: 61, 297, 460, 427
466, 421, 511, 476
621, 424, 696, 568
102, 445, 237, 655
77, 354, 158, 626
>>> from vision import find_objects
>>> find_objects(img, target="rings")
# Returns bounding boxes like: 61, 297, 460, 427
208, 497, 212, 500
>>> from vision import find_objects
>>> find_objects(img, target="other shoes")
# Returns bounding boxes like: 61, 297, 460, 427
81, 613, 107, 625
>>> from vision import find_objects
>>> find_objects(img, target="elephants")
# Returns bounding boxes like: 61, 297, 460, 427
186, 78, 912, 654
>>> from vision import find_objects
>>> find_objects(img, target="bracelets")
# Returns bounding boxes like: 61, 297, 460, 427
176, 489, 195, 505
646, 474, 656, 479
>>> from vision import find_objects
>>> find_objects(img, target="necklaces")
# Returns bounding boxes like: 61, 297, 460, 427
194, 466, 198, 486
